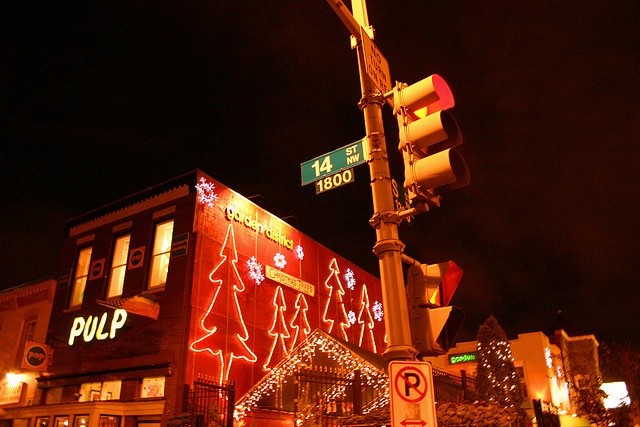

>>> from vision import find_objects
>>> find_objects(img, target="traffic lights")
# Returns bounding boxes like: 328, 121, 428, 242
409, 261, 465, 354
392, 74, 471, 195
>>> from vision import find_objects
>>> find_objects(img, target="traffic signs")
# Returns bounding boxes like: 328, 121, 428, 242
360, 26, 395, 108
300, 137, 369, 195
315, 168, 355, 194
